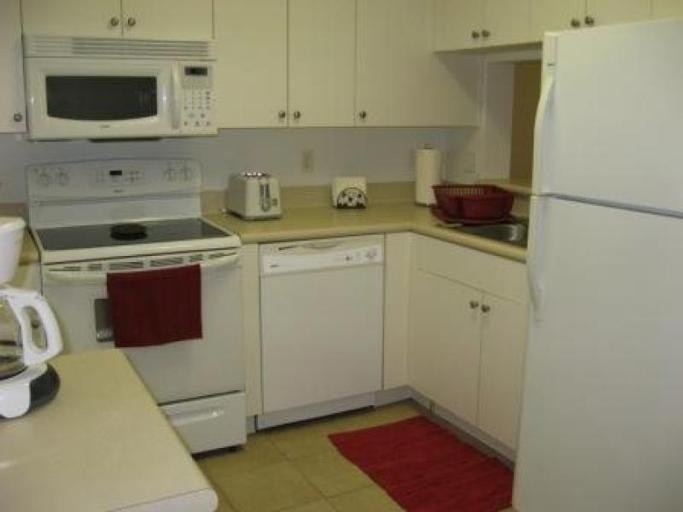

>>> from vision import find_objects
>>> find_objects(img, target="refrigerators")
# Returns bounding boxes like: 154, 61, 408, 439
510, 14, 682, 511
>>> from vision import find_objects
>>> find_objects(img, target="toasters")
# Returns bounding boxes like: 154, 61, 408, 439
226, 169, 283, 221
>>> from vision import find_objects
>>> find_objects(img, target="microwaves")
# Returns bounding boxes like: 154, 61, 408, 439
21, 35, 218, 143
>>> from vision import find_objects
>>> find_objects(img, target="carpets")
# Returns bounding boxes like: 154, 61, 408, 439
328, 414, 516, 512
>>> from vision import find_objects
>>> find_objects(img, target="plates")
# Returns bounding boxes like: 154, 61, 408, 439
428, 204, 515, 225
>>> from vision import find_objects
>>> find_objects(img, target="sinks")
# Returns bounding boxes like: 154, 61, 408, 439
445, 217, 528, 246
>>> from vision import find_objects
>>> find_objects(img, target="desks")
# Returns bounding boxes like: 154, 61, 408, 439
0, 349, 236, 512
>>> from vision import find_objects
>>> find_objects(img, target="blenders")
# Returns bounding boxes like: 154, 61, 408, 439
0, 217, 64, 421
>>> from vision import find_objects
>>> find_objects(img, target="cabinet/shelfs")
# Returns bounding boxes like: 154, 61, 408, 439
21, 0, 214, 40
429, 0, 681, 64
216, 0, 480, 129
2, 0, 26, 135
205, 196, 532, 467
